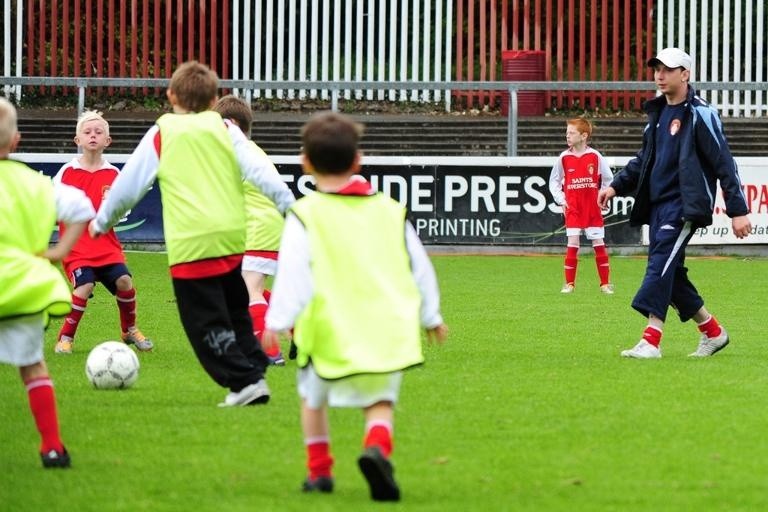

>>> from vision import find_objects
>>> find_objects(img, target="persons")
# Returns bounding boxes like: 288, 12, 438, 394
0, 98, 97, 469
52, 107, 155, 355
597, 46, 752, 358
213, 98, 296, 366
549, 117, 614, 295
84, 61, 296, 407
262, 112, 448, 501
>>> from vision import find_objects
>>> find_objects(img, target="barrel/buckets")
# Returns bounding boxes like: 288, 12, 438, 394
501, 50, 547, 118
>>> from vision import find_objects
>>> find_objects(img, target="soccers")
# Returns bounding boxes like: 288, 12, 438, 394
85, 341, 140, 389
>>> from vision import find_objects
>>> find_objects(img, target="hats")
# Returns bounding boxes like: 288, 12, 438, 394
648, 48, 692, 71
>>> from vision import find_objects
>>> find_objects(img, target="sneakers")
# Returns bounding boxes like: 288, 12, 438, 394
54, 335, 74, 354
217, 385, 266, 407
620, 338, 662, 359
560, 284, 574, 294
120, 326, 154, 351
40, 444, 70, 467
265, 352, 286, 366
303, 475, 332, 492
688, 324, 729, 358
359, 447, 401, 502
599, 284, 615, 294
